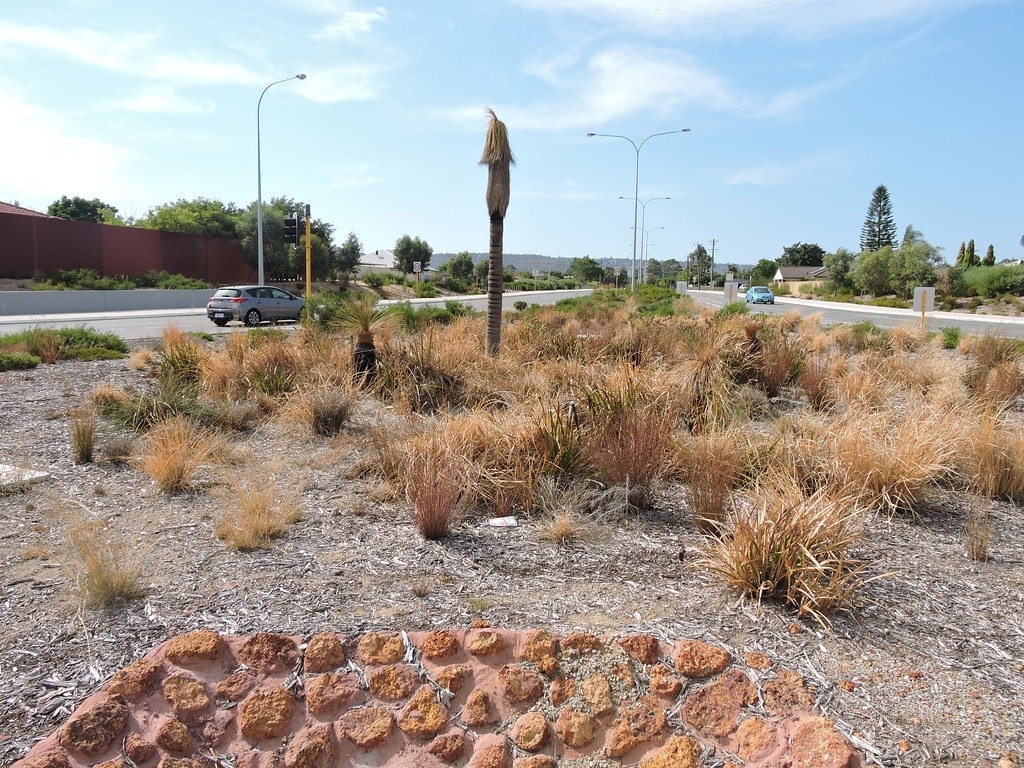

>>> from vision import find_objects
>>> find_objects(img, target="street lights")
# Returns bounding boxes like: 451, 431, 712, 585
629, 243, 654, 282
587, 128, 691, 292
256, 74, 307, 287
619, 197, 670, 283
631, 227, 665, 285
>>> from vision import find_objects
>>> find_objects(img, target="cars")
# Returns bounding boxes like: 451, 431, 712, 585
745, 287, 774, 304
206, 285, 306, 327
739, 284, 750, 293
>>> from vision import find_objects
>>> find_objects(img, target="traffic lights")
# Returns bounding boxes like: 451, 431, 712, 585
284, 212, 300, 248
